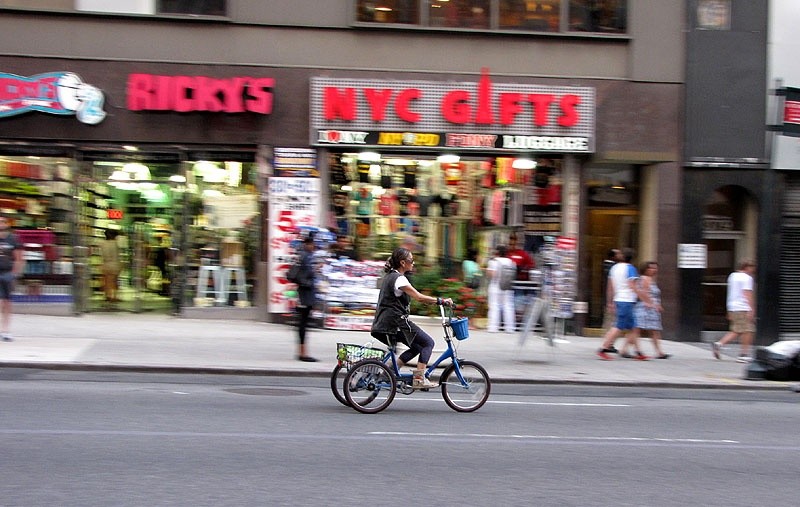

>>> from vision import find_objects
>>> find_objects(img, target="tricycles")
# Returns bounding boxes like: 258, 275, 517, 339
331, 301, 492, 413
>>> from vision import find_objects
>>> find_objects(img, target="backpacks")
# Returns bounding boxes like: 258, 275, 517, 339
493, 259, 517, 289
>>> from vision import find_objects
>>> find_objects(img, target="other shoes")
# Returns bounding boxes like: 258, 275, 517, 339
297, 355, 318, 362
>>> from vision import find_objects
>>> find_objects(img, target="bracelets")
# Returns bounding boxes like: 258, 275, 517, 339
437, 298, 444, 304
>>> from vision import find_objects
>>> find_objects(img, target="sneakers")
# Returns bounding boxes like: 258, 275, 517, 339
385, 359, 400, 377
735, 356, 751, 363
713, 341, 722, 359
413, 369, 439, 388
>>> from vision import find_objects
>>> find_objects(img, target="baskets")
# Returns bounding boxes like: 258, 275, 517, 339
337, 343, 387, 374
450, 317, 469, 340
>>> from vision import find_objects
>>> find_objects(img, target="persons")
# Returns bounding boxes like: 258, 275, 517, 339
462, 235, 536, 333
595, 248, 672, 361
295, 238, 322, 361
371, 247, 454, 389
711, 260, 757, 363
0, 217, 23, 342
101, 229, 123, 301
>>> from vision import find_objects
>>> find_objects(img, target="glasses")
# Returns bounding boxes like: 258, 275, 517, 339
404, 259, 414, 264
